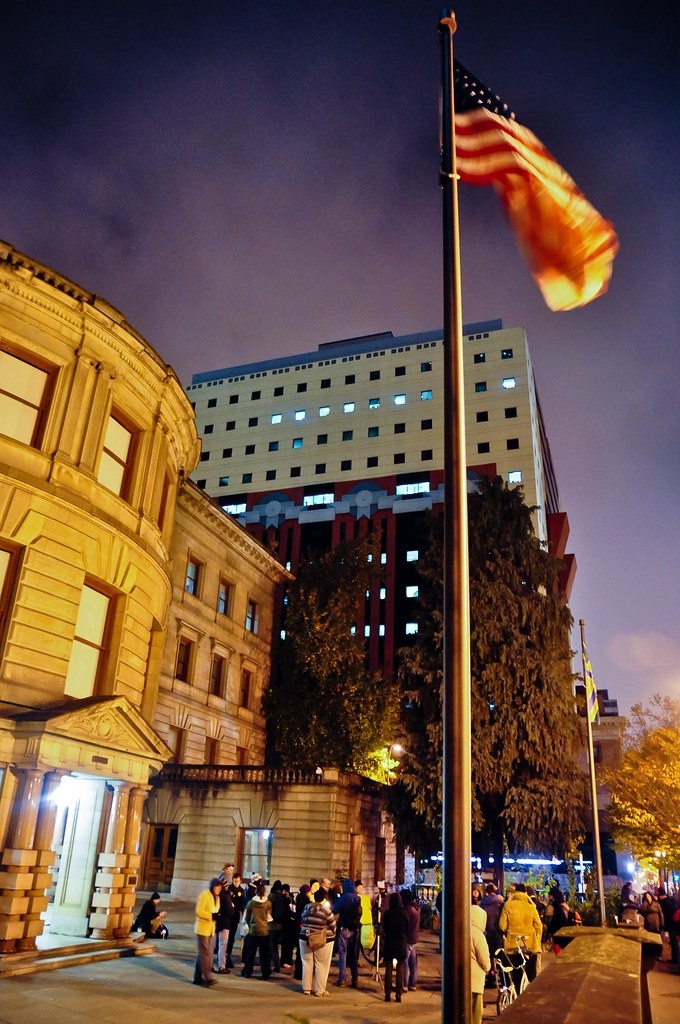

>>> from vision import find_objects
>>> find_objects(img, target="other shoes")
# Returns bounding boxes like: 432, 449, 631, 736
351, 982, 356, 986
338, 979, 346, 987
304, 991, 311, 995
261, 971, 273, 979
193, 977, 207, 984
403, 988, 409, 992
218, 968, 232, 974
207, 979, 218, 986
385, 996, 390, 1001
409, 986, 417, 991
315, 990, 329, 996
241, 971, 251, 978
395, 996, 402, 1002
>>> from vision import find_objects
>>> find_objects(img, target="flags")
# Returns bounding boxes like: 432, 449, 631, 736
581, 642, 600, 726
440, 63, 620, 311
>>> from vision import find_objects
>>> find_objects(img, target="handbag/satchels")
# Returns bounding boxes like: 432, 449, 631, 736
239, 908, 249, 936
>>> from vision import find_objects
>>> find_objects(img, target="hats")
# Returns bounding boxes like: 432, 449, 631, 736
251, 872, 262, 883
222, 863, 234, 870
151, 892, 160, 900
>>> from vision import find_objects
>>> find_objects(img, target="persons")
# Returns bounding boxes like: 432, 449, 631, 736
190, 863, 422, 1004
131, 892, 168, 938
434, 882, 680, 1024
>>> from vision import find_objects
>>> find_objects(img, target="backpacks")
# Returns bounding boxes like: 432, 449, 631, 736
307, 928, 326, 949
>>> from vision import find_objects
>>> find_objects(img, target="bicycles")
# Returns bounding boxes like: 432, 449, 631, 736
494, 935, 530, 1017
361, 924, 386, 968
